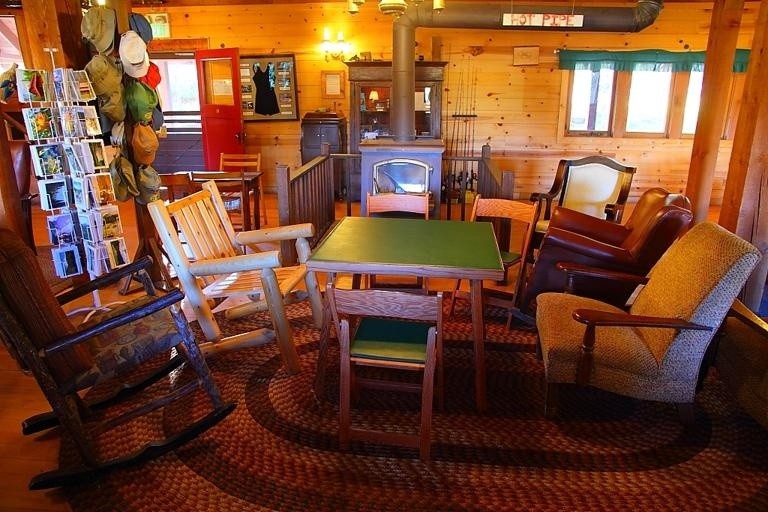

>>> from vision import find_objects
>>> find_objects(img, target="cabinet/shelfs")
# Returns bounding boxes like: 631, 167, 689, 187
298, 111, 348, 205
341, 61, 453, 207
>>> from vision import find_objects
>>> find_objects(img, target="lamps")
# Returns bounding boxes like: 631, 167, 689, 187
322, 39, 345, 62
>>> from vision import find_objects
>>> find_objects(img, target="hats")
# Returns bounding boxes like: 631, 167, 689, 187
81, 8, 116, 55
119, 30, 149, 78
101, 84, 126, 121
125, 82, 158, 126
136, 164, 161, 205
153, 106, 163, 130
129, 13, 152, 42
139, 63, 161, 89
132, 124, 159, 165
110, 122, 124, 144
110, 156, 140, 202
84, 55, 122, 96
